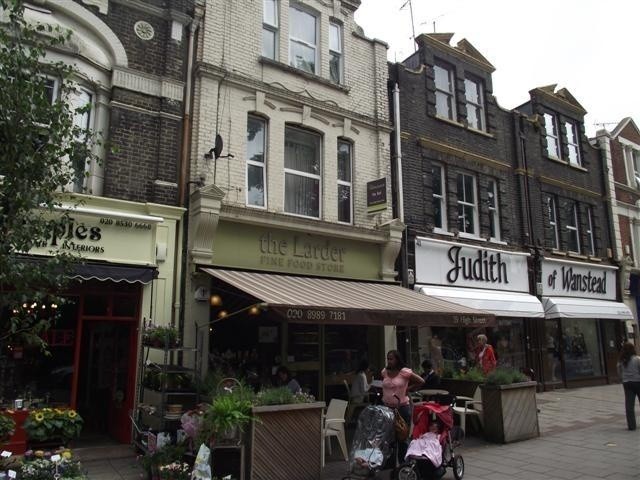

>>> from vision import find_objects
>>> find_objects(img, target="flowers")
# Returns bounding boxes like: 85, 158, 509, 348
25, 408, 83, 441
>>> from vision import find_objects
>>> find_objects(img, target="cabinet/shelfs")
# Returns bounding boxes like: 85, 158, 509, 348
130, 316, 200, 454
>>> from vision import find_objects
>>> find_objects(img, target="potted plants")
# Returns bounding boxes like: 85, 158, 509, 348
0, 414, 16, 442
143, 324, 177, 348
209, 395, 263, 439
162, 375, 192, 413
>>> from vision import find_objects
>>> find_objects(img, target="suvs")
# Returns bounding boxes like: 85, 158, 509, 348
421, 344, 461, 373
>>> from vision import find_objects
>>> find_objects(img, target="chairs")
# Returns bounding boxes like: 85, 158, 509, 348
323, 398, 349, 466
453, 386, 484, 440
207, 378, 247, 480
343, 379, 377, 424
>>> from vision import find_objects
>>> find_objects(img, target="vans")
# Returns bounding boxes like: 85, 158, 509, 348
324, 348, 363, 368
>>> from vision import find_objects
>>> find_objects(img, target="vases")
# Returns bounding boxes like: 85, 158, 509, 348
25, 433, 64, 450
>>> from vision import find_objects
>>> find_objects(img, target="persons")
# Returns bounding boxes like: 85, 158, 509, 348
616, 341, 640, 430
497, 335, 514, 368
473, 334, 497, 377
349, 350, 441, 468
275, 361, 302, 395
429, 334, 442, 374
545, 323, 587, 383
237, 345, 268, 392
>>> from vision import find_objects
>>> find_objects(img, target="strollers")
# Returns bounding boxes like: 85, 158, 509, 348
345, 390, 406, 479
395, 391, 468, 479
